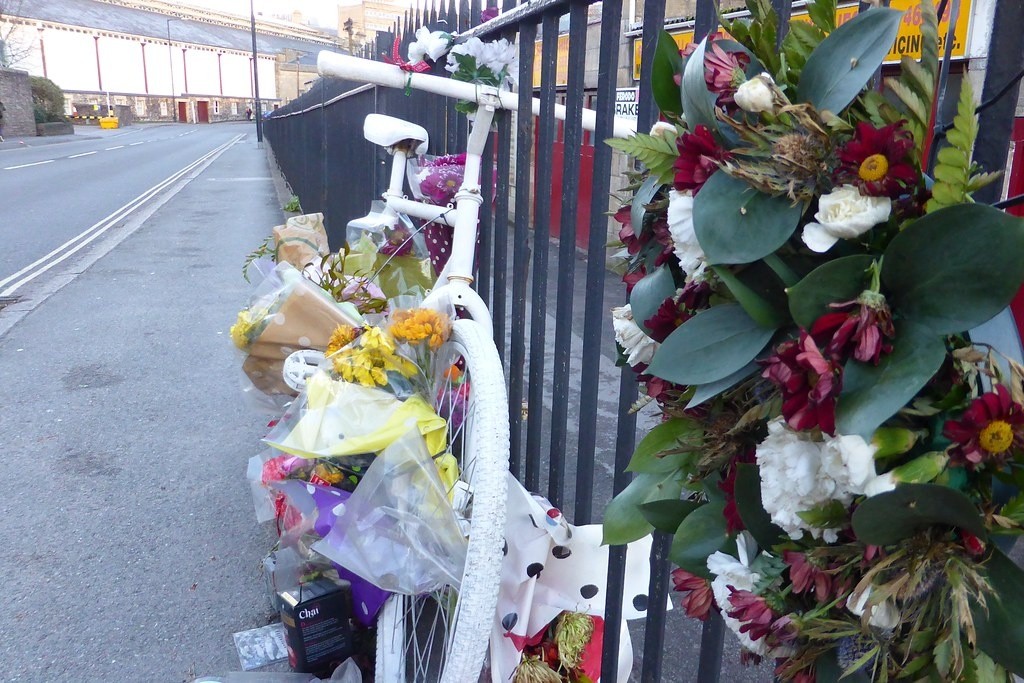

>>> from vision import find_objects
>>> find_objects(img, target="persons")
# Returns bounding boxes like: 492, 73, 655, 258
246, 107, 252, 121
0, 102, 6, 142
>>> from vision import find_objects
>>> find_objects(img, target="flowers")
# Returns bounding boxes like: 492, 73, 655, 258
232, 152, 673, 683
602, 0, 1024, 683
445, 37, 520, 132
407, 24, 456, 63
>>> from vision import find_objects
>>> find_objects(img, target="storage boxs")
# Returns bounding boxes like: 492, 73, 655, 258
276, 576, 355, 672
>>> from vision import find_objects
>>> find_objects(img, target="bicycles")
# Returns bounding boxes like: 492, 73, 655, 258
283, 49, 640, 683
